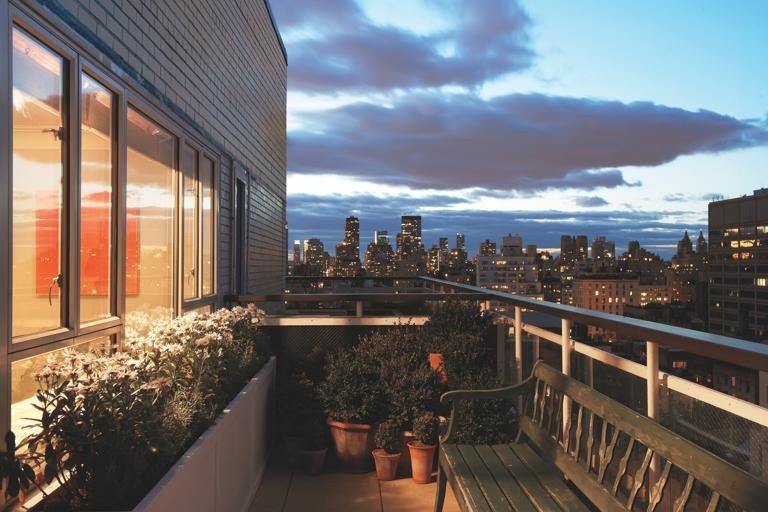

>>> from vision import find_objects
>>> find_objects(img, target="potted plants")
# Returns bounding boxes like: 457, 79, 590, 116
279, 295, 517, 482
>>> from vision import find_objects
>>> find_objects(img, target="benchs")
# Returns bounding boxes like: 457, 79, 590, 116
434, 358, 768, 511
